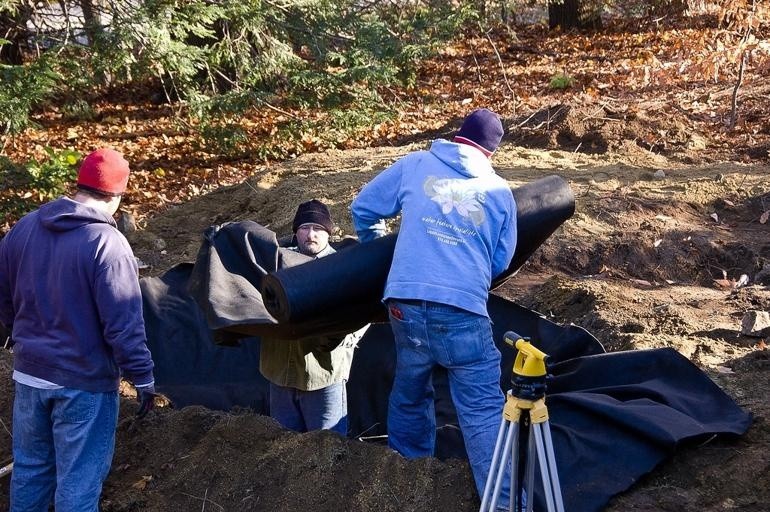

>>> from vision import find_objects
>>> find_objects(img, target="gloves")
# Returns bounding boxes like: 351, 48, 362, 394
135, 392, 154, 420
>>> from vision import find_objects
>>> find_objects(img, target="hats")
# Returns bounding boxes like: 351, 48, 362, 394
293, 200, 332, 236
77, 148, 130, 193
453, 108, 504, 158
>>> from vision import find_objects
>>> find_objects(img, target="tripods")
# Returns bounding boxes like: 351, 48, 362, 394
478, 396, 566, 512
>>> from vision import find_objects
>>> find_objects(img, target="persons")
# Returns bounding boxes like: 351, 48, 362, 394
349, 108, 535, 510
0, 143, 157, 510
201, 198, 356, 437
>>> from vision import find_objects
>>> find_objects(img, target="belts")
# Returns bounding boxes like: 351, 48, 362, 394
398, 298, 422, 306
